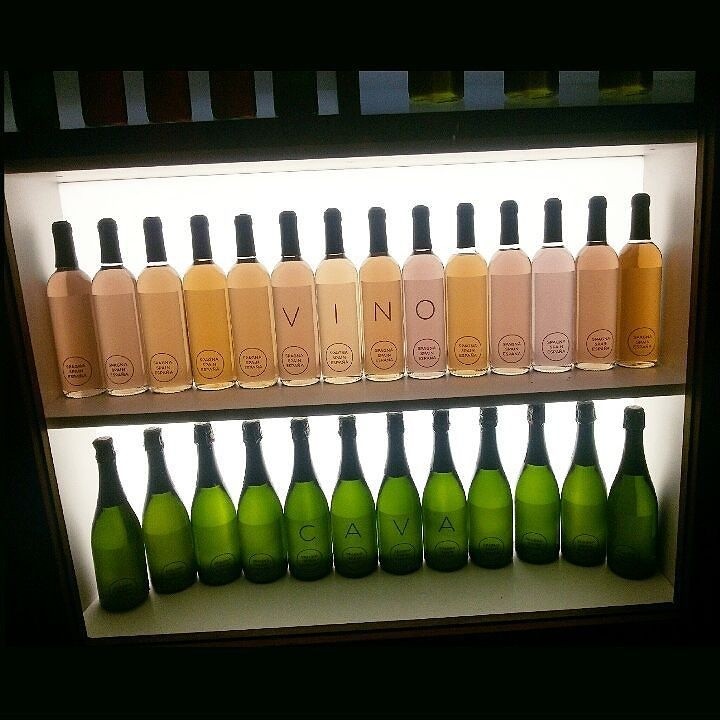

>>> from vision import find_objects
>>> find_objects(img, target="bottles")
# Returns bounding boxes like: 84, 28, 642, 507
422, 409, 469, 572
143, 428, 197, 595
562, 402, 608, 567
91, 218, 148, 396
314, 209, 362, 384
376, 411, 422, 575
619, 194, 662, 368
468, 407, 513, 569
331, 415, 377, 579
489, 200, 533, 375
576, 196, 620, 370
135, 217, 193, 394
227, 214, 277, 388
405, 205, 446, 380
284, 419, 333, 581
92, 436, 149, 614
183, 215, 235, 391
608, 405, 658, 580
359, 207, 405, 381
191, 423, 242, 586
269, 211, 316, 386
445, 203, 488, 377
516, 402, 561, 565
46, 221, 106, 398
237, 421, 287, 584
532, 198, 577, 373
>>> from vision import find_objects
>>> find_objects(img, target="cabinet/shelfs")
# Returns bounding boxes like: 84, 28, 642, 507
0, 0, 720, 720
